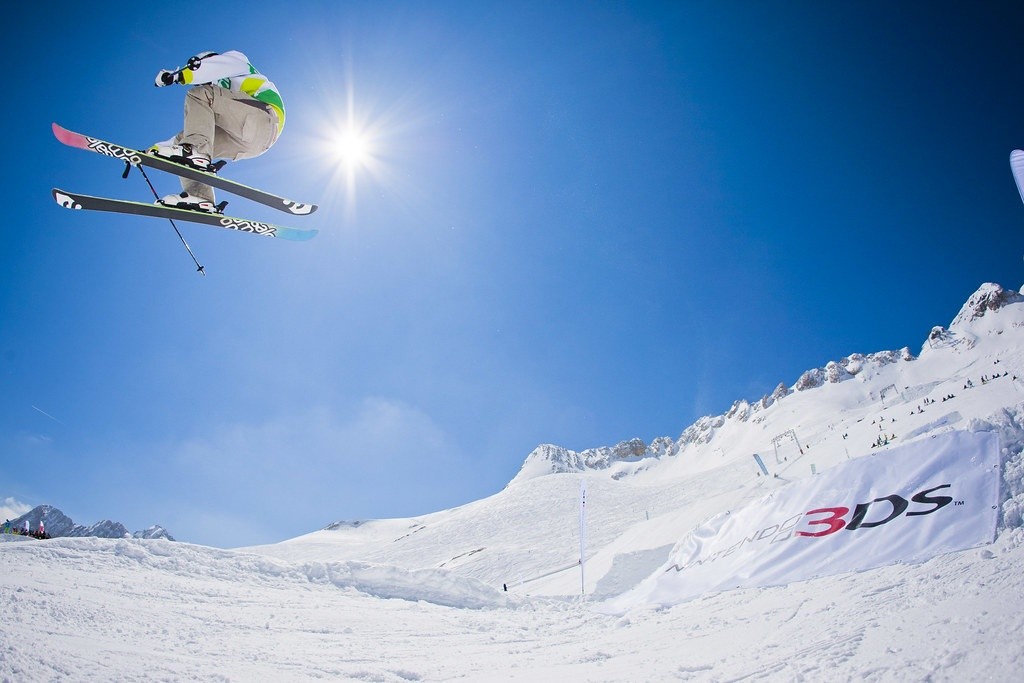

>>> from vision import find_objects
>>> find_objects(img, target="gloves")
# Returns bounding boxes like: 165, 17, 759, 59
155, 66, 180, 88
154, 135, 180, 146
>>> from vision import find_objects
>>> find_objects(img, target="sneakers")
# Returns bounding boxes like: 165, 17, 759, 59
154, 193, 216, 213
148, 144, 211, 172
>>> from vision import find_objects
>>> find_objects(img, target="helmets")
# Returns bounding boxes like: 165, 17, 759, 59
197, 50, 218, 59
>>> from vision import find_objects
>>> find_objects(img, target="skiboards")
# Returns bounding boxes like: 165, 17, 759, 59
46, 116, 324, 245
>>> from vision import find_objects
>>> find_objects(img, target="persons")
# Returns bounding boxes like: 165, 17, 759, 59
1, 519, 52, 540
147, 51, 285, 211
